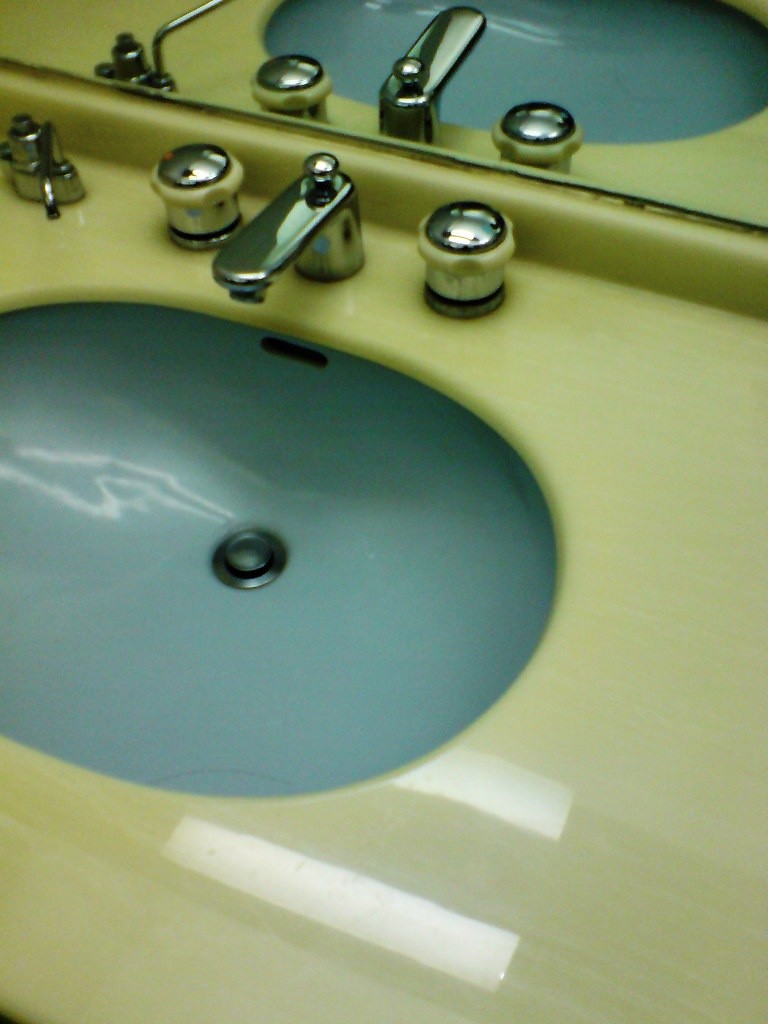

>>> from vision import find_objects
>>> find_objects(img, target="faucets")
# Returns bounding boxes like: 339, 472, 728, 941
211, 153, 368, 306
379, 5, 486, 147
37, 115, 85, 223
142, 2, 232, 92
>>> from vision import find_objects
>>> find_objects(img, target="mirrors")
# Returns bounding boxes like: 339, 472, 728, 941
0, 0, 768, 239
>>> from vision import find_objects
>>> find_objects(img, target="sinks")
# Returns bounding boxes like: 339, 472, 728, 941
252, 0, 768, 150
1, 286, 567, 802
